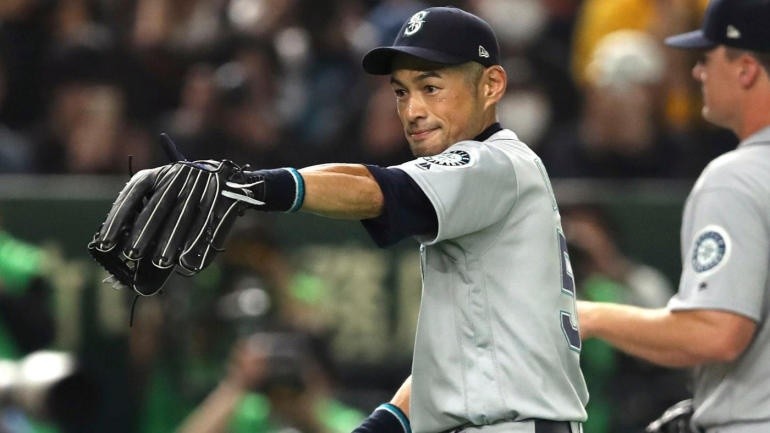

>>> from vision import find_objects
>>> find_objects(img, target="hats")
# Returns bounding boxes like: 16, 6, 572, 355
361, 7, 499, 75
663, 0, 770, 54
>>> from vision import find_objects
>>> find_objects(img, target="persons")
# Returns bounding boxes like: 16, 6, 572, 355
0, 0, 738, 433
87, 5, 589, 433
574, 0, 770, 433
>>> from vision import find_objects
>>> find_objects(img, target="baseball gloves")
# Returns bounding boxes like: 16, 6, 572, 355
86, 159, 251, 298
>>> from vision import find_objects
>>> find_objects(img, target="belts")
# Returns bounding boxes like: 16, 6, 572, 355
535, 419, 572, 433
